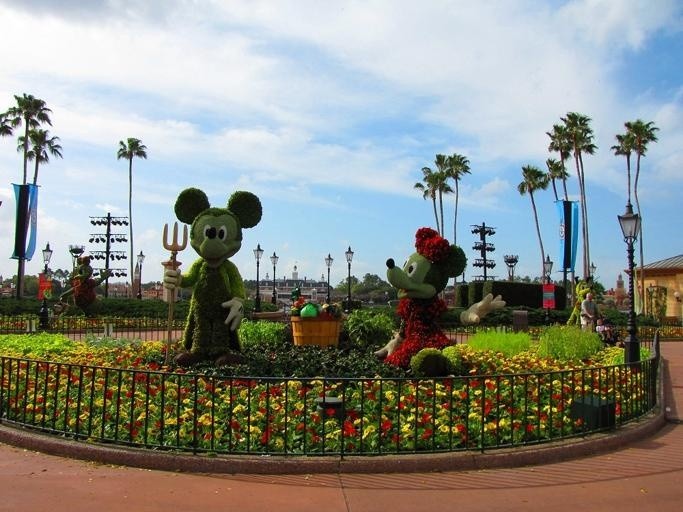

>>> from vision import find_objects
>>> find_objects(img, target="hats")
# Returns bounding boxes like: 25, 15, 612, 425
586, 293, 593, 298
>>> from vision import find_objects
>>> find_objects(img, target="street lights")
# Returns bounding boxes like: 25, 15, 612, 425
68, 244, 86, 272
135, 250, 145, 299
615, 198, 645, 374
37, 240, 56, 328
501, 252, 519, 283
86, 209, 130, 298
154, 282, 162, 301
468, 219, 496, 280
542, 252, 554, 327
586, 261, 598, 282
251, 241, 355, 313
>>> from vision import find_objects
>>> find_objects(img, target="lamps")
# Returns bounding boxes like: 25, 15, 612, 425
671, 289, 682, 303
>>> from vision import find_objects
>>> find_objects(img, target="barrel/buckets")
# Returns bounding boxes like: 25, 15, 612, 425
290, 315, 341, 350
311, 397, 345, 419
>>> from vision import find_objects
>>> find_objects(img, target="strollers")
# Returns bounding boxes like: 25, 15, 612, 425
589, 312, 624, 349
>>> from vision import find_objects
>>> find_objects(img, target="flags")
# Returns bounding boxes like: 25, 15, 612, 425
542, 284, 556, 309
36, 273, 52, 300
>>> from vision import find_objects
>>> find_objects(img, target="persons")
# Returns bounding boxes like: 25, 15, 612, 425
579, 292, 594, 333
595, 318, 610, 340
